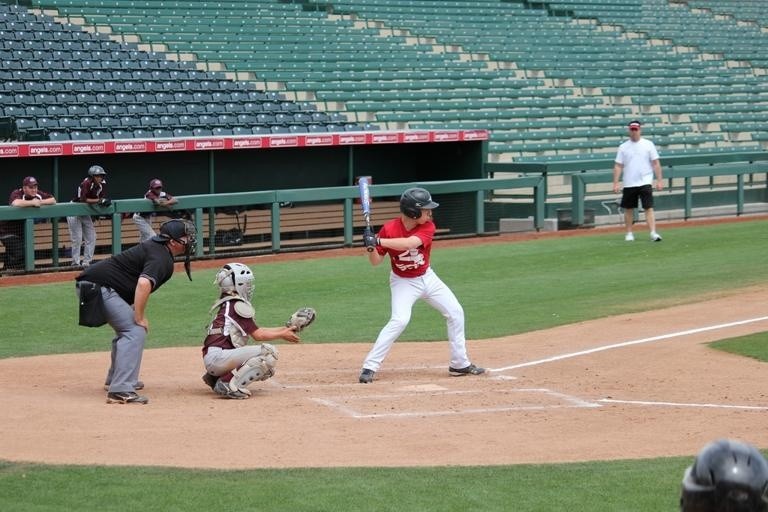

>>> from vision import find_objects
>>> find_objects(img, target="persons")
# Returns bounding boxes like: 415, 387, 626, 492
609, 117, 666, 245
7, 175, 57, 269
131, 177, 179, 245
357, 186, 489, 384
70, 218, 198, 407
64, 164, 111, 271
200, 260, 306, 399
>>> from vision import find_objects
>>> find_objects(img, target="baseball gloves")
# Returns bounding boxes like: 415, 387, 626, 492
286, 308, 315, 331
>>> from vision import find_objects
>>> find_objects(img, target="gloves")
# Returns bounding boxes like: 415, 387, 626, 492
364, 231, 380, 246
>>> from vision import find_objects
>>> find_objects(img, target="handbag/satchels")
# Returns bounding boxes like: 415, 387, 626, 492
79, 284, 108, 327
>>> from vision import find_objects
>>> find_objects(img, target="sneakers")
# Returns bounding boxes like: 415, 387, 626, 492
649, 232, 661, 242
625, 232, 635, 241
449, 364, 485, 376
203, 373, 249, 399
359, 369, 374, 383
104, 381, 148, 405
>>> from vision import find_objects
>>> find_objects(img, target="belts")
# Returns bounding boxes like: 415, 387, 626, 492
76, 275, 104, 284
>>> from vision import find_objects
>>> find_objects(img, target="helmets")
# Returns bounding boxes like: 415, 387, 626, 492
400, 188, 439, 219
215, 262, 255, 300
88, 165, 106, 176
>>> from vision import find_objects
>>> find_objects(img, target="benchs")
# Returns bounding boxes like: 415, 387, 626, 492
33, 201, 450, 250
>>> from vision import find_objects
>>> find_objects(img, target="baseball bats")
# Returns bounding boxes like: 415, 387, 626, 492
358, 177, 374, 253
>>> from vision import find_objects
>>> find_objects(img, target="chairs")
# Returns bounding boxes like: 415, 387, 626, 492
0, 0, 768, 163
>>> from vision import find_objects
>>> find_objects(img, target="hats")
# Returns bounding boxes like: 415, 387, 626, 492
24, 177, 37, 186
630, 121, 640, 131
152, 219, 198, 242
150, 179, 162, 189
